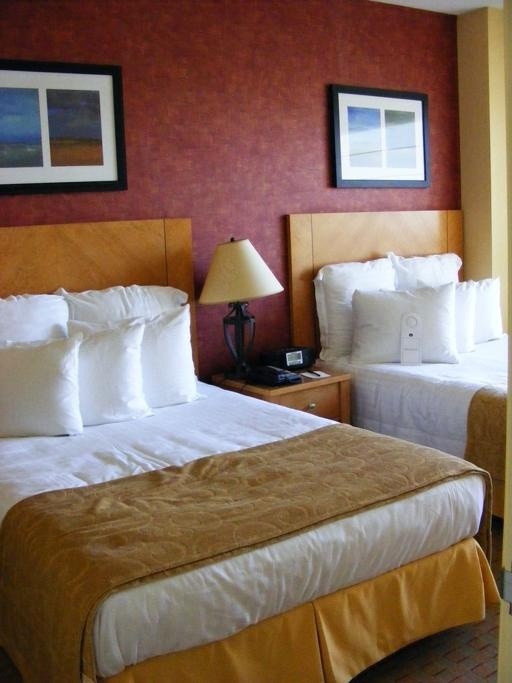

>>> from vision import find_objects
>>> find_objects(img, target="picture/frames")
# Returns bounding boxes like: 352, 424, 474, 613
329, 84, 430, 190
0, 58, 128, 196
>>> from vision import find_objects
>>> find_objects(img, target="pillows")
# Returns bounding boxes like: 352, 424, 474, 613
1, 285, 206, 439
313, 254, 503, 363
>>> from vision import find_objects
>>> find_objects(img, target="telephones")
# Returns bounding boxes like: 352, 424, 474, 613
247, 365, 294, 387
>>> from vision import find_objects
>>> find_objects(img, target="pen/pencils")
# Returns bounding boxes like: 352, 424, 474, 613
306, 369, 320, 377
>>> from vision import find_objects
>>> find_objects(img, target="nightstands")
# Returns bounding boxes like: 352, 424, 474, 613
212, 367, 353, 426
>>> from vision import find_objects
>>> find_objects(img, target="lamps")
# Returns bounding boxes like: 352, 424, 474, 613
198, 237, 285, 380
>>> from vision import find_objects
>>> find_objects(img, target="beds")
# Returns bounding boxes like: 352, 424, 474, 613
287, 209, 511, 520
1, 218, 501, 683
